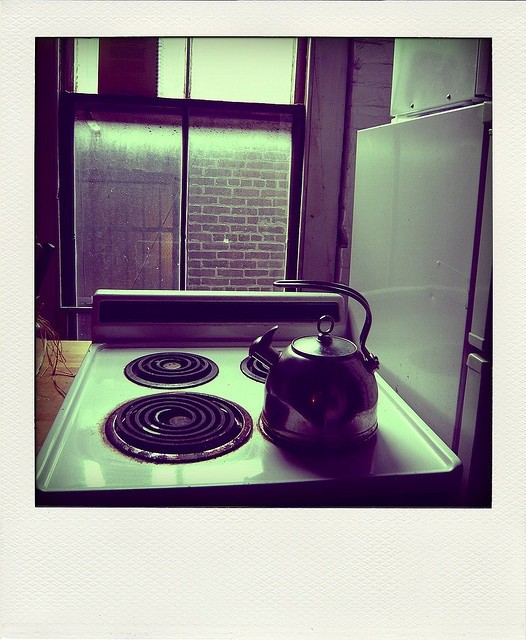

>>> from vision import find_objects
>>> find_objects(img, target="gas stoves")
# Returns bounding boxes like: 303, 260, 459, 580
35, 286, 465, 509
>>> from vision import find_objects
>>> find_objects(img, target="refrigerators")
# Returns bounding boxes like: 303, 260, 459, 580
347, 98, 492, 495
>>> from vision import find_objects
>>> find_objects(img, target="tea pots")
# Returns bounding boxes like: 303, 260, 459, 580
246, 278, 383, 454
